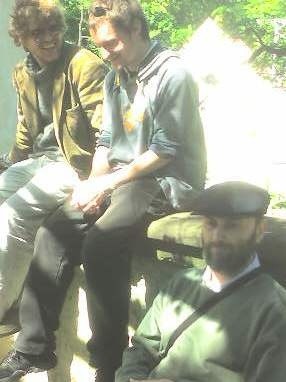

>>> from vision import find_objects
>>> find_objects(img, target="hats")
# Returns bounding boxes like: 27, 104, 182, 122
189, 181, 272, 218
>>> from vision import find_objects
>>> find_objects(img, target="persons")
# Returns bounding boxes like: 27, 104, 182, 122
114, 180, 286, 382
0, 0, 113, 324
0, 0, 207, 381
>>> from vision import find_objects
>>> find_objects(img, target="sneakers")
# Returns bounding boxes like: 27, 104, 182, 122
0, 348, 58, 382
94, 364, 120, 382
